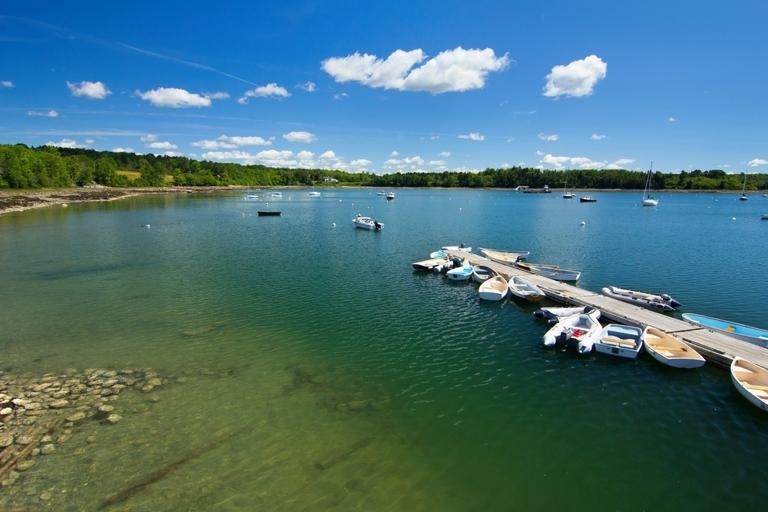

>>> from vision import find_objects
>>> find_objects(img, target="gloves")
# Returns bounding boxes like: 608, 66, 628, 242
680, 310, 767, 348
351, 215, 384, 232
729, 353, 768, 410
601, 285, 682, 313
447, 264, 547, 303
531, 301, 708, 372
579, 194, 597, 203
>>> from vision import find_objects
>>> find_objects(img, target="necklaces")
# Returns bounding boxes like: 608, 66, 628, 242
641, 160, 660, 207
